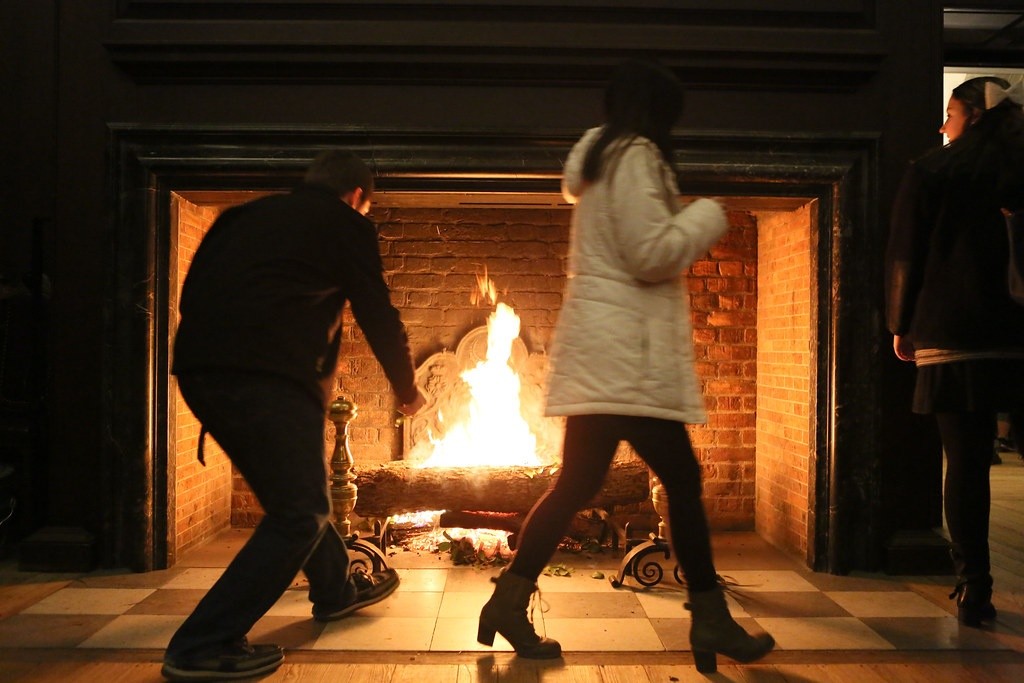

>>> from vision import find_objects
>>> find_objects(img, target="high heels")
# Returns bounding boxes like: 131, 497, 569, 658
681, 586, 776, 674
477, 571, 562, 661
952, 578, 999, 626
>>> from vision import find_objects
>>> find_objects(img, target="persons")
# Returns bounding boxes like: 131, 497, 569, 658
478, 59, 774, 673
885, 77, 1023, 625
159, 149, 426, 683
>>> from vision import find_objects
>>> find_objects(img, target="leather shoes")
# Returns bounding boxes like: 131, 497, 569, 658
312, 566, 399, 619
161, 635, 284, 680
